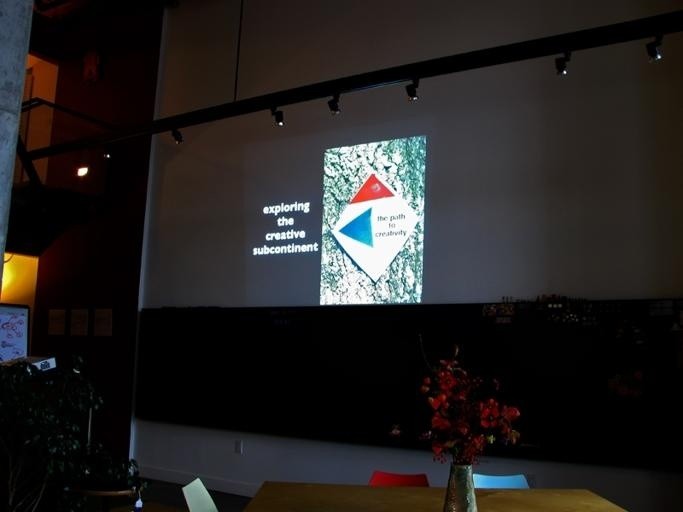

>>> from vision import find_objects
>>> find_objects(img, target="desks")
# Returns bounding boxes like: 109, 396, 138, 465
244, 479, 633, 512
65, 481, 146, 512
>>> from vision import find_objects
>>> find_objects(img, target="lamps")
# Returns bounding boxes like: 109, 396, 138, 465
554, 29, 665, 79
171, 129, 184, 145
269, 79, 423, 128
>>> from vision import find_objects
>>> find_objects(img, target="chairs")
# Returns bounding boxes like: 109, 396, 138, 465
181, 477, 220, 512
368, 471, 532, 489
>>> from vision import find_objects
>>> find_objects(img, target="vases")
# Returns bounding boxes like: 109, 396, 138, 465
442, 463, 479, 512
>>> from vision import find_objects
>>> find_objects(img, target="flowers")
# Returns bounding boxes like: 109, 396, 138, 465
408, 343, 521, 465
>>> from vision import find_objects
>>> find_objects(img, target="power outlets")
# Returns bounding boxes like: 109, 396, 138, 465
234, 440, 244, 455
